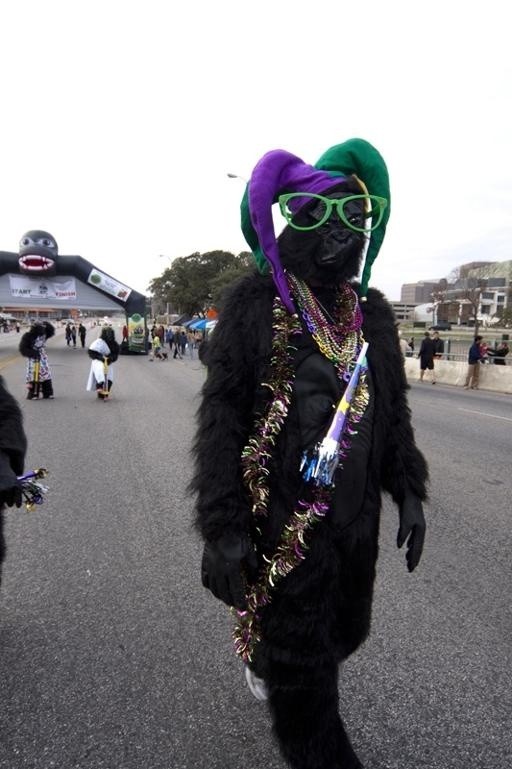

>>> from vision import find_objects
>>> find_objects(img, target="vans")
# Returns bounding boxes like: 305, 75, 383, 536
428, 322, 452, 331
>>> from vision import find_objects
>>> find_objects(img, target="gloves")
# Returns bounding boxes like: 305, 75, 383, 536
395, 495, 427, 574
202, 537, 260, 611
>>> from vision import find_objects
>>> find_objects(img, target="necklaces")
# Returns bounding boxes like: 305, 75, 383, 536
284, 270, 363, 364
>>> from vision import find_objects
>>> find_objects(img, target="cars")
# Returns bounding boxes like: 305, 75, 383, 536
61, 318, 75, 325
0, 313, 17, 323
96, 318, 112, 325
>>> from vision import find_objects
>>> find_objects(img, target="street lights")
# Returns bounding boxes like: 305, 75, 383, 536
159, 254, 172, 263
226, 171, 247, 186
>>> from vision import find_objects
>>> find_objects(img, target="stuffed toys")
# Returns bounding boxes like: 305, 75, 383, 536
181, 139, 430, 768
0, 375, 25, 571
20, 320, 55, 399
86, 326, 120, 398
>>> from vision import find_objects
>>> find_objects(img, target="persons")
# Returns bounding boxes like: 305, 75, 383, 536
122, 325, 128, 342
399, 327, 508, 390
16, 320, 20, 332
65, 323, 87, 348
150, 314, 216, 362
2, 321, 10, 332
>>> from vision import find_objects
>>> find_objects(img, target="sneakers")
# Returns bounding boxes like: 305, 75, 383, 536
418, 378, 437, 385
31, 395, 56, 400
243, 663, 272, 706
463, 385, 480, 391
